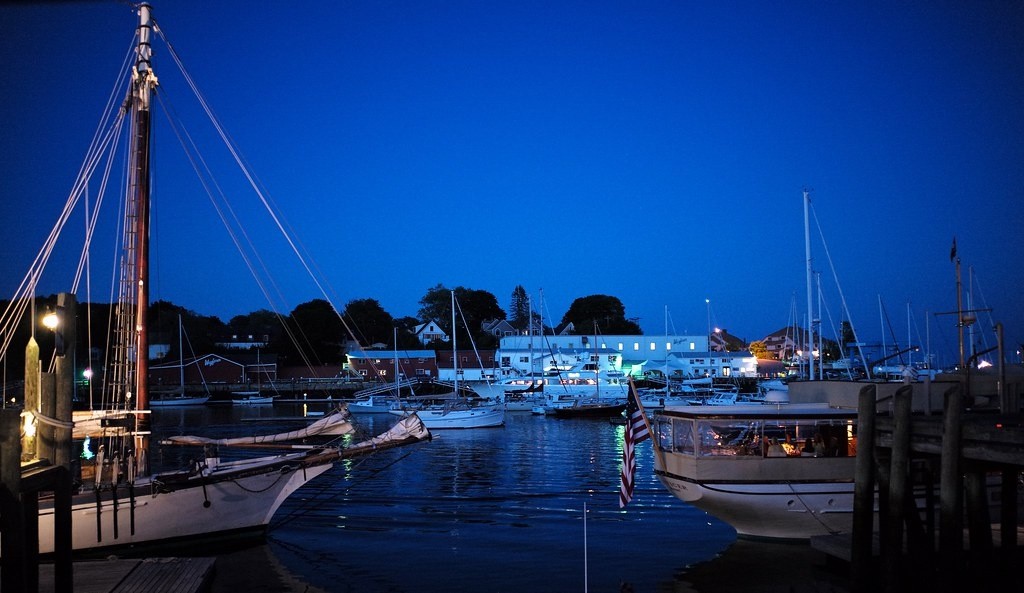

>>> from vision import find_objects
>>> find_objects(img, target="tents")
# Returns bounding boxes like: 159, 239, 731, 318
631, 358, 691, 378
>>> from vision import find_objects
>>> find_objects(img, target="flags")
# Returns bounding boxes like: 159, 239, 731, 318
619, 382, 651, 508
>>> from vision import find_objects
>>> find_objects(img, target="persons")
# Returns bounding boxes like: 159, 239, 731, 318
800, 433, 840, 457
759, 432, 796, 458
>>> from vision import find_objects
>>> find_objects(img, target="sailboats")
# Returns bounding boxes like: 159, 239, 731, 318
148, 311, 214, 406
230, 347, 274, 405
0, 0, 433, 551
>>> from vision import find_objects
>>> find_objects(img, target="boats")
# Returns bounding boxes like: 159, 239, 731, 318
334, 187, 1024, 539
